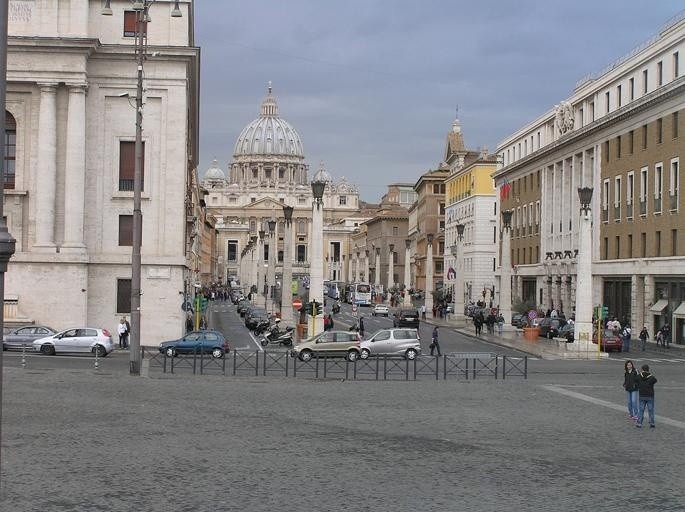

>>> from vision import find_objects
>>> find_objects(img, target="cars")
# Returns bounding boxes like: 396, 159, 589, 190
2, 323, 60, 354
370, 303, 389, 317
358, 324, 423, 362
30, 327, 113, 357
156, 329, 230, 359
228, 279, 364, 362
446, 301, 623, 353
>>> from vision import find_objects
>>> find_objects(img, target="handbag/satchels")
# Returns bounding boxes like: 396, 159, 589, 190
626, 333, 631, 339
429, 343, 435, 348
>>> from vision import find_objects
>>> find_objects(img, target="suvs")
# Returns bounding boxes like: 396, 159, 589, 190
390, 308, 419, 329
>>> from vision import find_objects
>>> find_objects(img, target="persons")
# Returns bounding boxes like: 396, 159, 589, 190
118, 319, 128, 350
198, 315, 208, 330
202, 288, 228, 303
622, 360, 642, 420
419, 300, 575, 337
360, 316, 365, 337
430, 326, 442, 356
634, 363, 657, 428
328, 314, 335, 329
332, 300, 338, 313
186, 315, 194, 333
123, 316, 131, 348
592, 315, 671, 351
324, 315, 330, 331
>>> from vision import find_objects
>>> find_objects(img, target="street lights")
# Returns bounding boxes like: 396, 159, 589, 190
494, 209, 516, 332
96, 1, 184, 377
571, 184, 599, 352
265, 217, 277, 314
237, 226, 265, 292
340, 242, 395, 300
424, 232, 435, 321
306, 174, 328, 338
279, 202, 295, 324
447, 222, 467, 325
403, 238, 412, 308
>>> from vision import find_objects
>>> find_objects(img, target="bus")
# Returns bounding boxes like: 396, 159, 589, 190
344, 282, 372, 307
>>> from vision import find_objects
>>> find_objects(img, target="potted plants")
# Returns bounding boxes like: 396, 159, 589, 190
512, 296, 542, 340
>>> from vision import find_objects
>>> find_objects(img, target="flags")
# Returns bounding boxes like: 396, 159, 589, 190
500, 176, 511, 202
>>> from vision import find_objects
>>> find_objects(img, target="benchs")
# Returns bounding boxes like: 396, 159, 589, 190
553, 337, 568, 347
515, 329, 524, 336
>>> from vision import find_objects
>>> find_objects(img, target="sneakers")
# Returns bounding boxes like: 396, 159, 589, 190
627, 414, 638, 420
636, 425, 641, 429
649, 423, 655, 432
